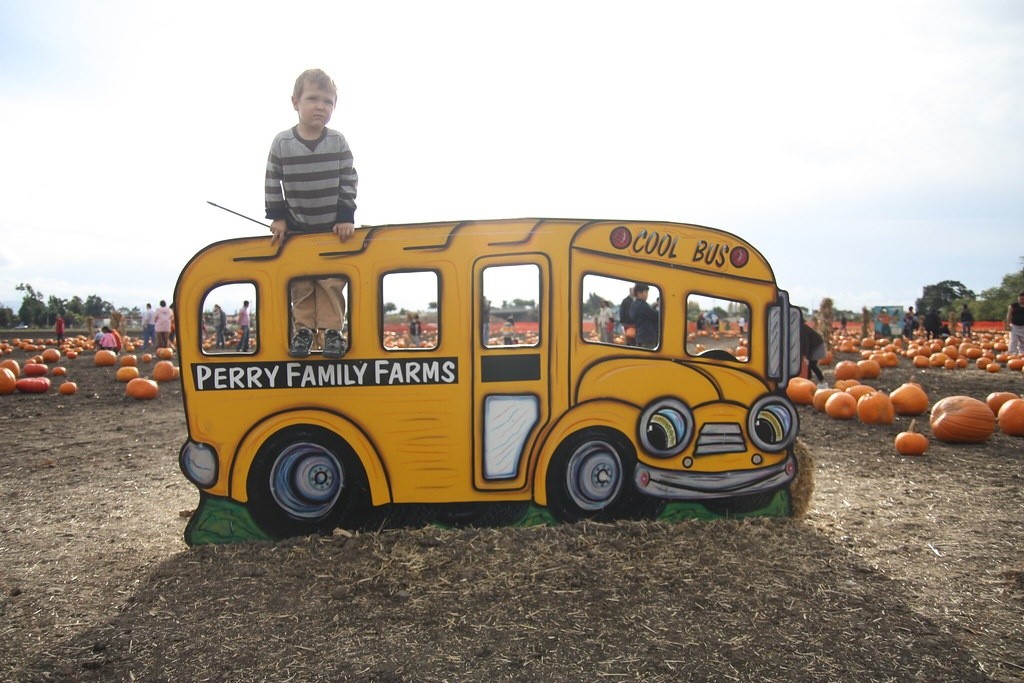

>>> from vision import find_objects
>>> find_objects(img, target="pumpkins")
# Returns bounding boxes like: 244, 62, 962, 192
486, 330, 538, 345
588, 329, 625, 345
0, 332, 256, 399
689, 330, 748, 362
786, 324, 1024, 454
383, 331, 438, 348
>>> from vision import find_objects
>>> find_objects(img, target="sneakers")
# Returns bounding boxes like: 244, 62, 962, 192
323, 329, 347, 358
289, 327, 313, 357
818, 383, 828, 389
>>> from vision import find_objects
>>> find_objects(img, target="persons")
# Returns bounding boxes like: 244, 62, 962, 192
739, 315, 745, 334
54, 314, 65, 349
504, 318, 514, 344
95, 326, 122, 357
922, 307, 951, 341
903, 306, 918, 341
807, 316, 829, 389
154, 300, 175, 349
696, 312, 720, 332
407, 314, 421, 347
839, 313, 849, 336
630, 284, 659, 349
598, 301, 616, 343
960, 304, 971, 337
265, 69, 358, 357
212, 304, 226, 348
237, 301, 250, 351
1007, 293, 1024, 354
620, 288, 636, 347
140, 303, 155, 349
